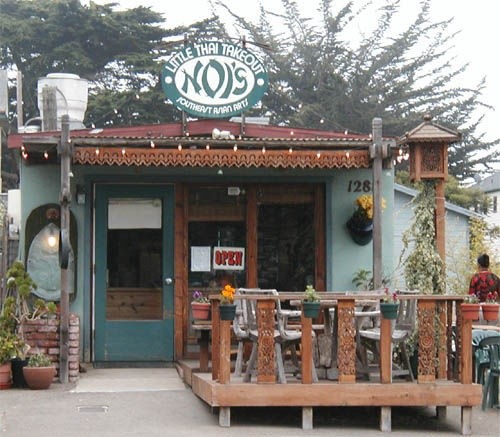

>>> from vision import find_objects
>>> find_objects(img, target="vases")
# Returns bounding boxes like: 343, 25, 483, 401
219, 305, 237, 320
482, 304, 499, 321
346, 219, 373, 246
460, 303, 480, 320
190, 302, 211, 320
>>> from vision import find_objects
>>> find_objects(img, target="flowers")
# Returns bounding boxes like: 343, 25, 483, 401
221, 285, 236, 304
192, 290, 211, 303
487, 291, 498, 304
349, 194, 386, 227
464, 291, 481, 304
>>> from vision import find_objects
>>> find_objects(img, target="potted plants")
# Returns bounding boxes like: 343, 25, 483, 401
22, 353, 56, 389
0, 337, 17, 390
302, 285, 321, 318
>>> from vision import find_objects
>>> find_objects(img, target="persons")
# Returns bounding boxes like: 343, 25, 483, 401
470, 253, 500, 305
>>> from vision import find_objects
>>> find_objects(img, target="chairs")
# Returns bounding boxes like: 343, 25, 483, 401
233, 287, 421, 383
471, 329, 500, 410
